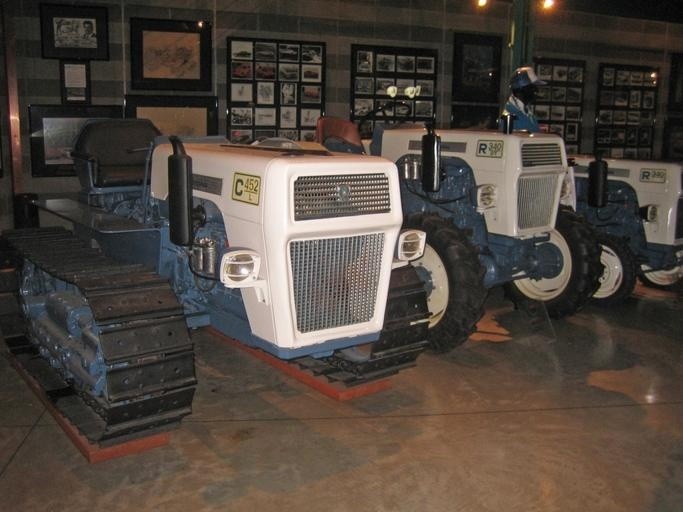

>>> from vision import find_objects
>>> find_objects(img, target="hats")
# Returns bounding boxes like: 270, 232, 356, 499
508, 65, 548, 89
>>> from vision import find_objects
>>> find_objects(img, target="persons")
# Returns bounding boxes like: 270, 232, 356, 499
499, 66, 549, 132
82, 21, 95, 38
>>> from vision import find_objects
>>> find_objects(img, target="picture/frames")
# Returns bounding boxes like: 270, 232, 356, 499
59, 58, 91, 105
123, 94, 219, 138
530, 56, 683, 161
129, 15, 213, 92
450, 31, 504, 130
350, 42, 439, 140
38, 2, 111, 62
28, 103, 124, 178
226, 36, 327, 144
